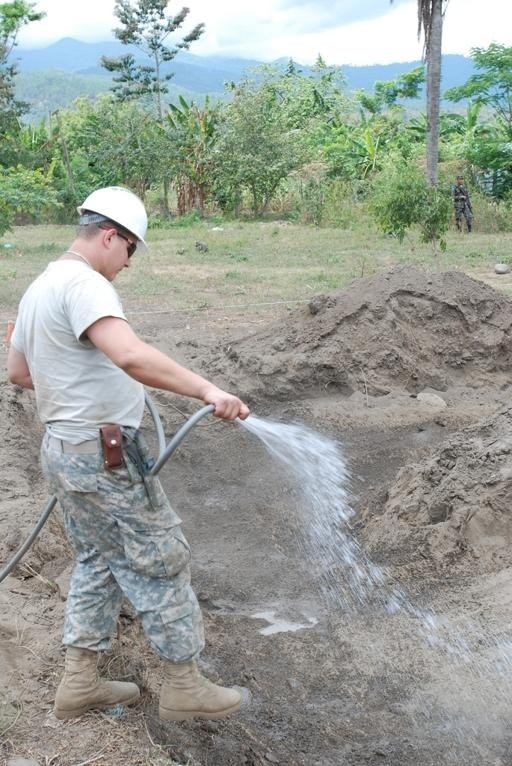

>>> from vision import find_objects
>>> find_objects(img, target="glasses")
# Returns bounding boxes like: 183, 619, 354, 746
97, 224, 136, 258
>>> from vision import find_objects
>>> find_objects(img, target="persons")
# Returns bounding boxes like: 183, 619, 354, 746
5, 184, 253, 719
453, 174, 474, 232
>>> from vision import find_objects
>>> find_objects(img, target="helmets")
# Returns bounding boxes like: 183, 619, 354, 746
76, 186, 149, 250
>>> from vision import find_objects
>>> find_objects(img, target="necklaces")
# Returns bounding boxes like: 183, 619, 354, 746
64, 249, 90, 267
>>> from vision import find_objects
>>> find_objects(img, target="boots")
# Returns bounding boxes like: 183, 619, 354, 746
53, 646, 140, 719
158, 658, 243, 721
